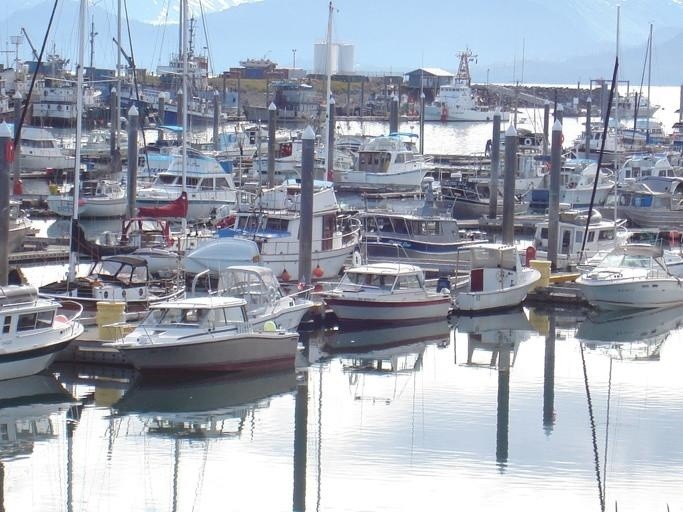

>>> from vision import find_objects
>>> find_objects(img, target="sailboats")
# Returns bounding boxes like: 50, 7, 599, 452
0, 118, 85, 379
0, 1, 682, 327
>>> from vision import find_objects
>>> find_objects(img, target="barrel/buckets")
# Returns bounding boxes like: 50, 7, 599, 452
529, 308, 547, 335
95, 300, 127, 341
48, 183, 58, 194
436, 276, 451, 293
94, 376, 126, 408
530, 259, 551, 289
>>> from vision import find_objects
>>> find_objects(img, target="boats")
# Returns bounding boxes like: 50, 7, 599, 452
108, 295, 302, 382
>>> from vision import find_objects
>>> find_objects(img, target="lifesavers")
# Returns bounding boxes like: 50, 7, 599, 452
5, 140, 14, 162
